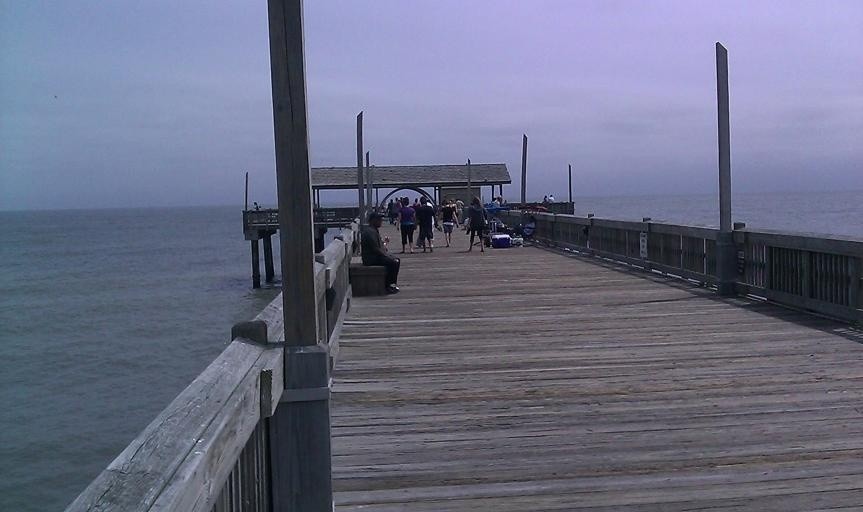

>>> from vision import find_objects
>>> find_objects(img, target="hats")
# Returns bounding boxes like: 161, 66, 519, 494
369, 212, 385, 219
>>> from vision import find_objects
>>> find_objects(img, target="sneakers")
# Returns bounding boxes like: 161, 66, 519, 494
387, 284, 399, 293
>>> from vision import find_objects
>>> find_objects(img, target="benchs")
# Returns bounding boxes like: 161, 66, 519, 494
350, 256, 387, 298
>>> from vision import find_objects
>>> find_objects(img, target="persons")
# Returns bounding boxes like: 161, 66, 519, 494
360, 212, 401, 293
382, 195, 536, 253
252, 202, 261, 211
548, 195, 554, 203
542, 196, 548, 204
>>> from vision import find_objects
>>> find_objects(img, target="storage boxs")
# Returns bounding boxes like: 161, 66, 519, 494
492, 234, 511, 248
